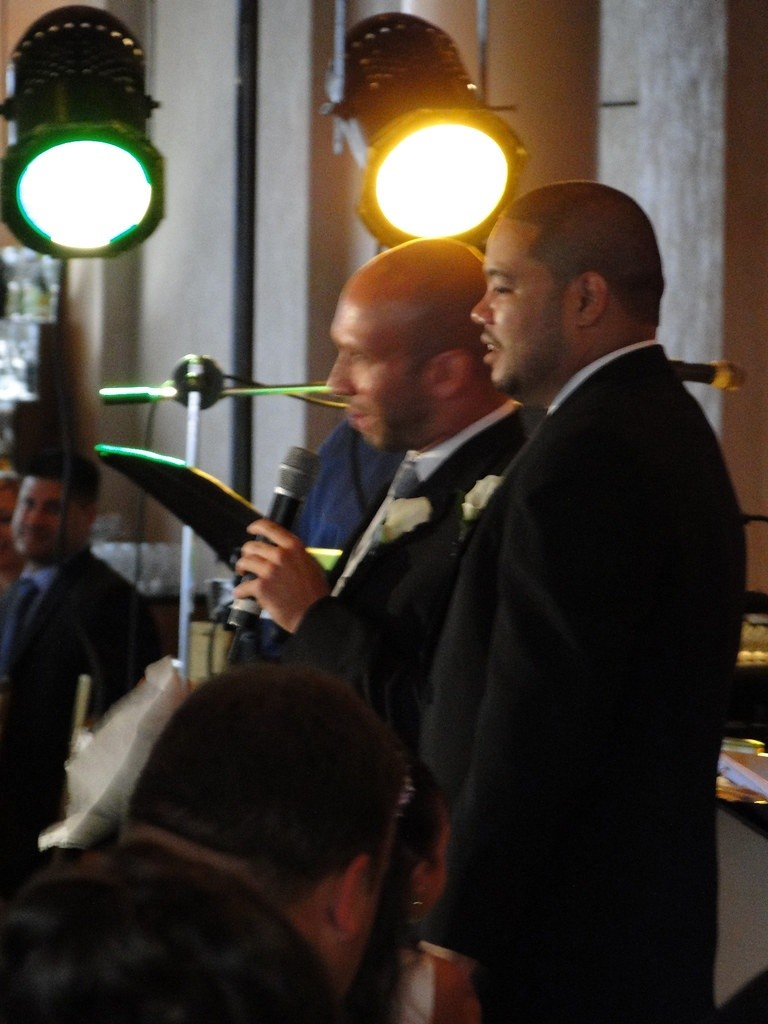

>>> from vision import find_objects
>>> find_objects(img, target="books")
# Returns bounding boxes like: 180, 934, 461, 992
717, 750, 768, 799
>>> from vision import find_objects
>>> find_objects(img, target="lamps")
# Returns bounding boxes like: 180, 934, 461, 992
329, 12, 530, 249
0, 5, 163, 258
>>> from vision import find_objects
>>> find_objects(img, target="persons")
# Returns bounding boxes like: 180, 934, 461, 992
0, 664, 477, 1024
228, 238, 541, 750
0, 453, 167, 896
422, 180, 748, 1024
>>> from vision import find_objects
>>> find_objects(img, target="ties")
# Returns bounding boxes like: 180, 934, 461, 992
0, 579, 38, 677
394, 468, 420, 500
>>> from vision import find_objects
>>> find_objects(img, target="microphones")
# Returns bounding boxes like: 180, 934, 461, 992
226, 447, 322, 662
669, 358, 749, 391
